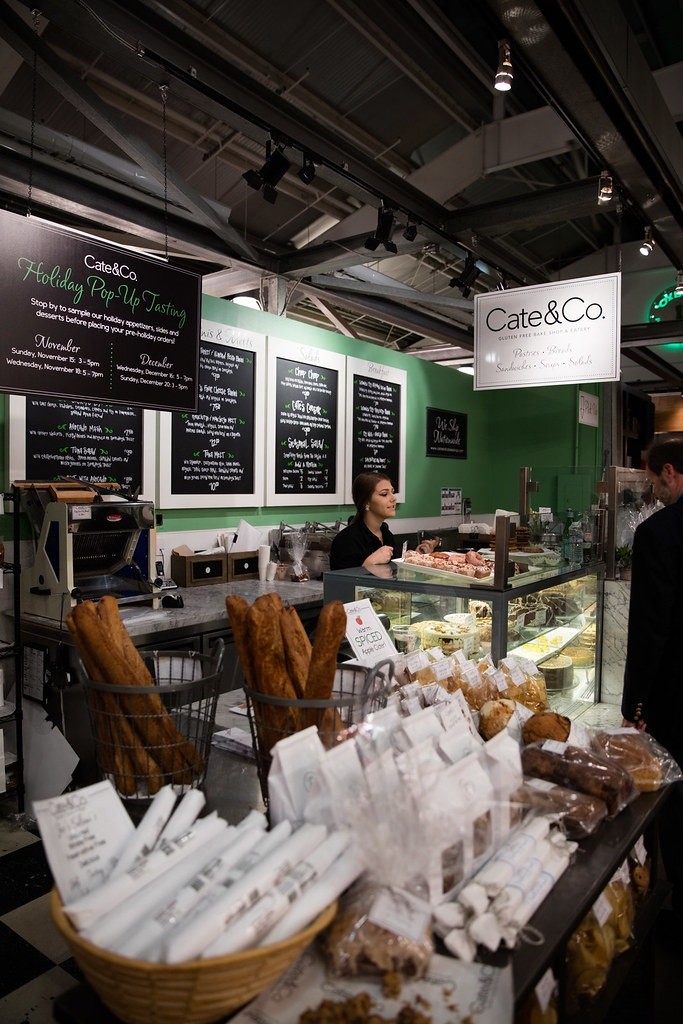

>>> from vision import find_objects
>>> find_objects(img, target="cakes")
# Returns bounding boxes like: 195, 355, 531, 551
407, 574, 597, 692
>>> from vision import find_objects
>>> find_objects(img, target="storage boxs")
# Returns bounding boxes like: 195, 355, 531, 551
171, 544, 227, 588
227, 549, 260, 581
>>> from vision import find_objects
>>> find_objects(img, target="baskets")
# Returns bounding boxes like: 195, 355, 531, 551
51, 880, 338, 1024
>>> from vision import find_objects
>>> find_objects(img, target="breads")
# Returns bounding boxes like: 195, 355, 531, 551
296, 657, 665, 1024
226, 591, 349, 755
489, 526, 545, 553
65, 596, 202, 796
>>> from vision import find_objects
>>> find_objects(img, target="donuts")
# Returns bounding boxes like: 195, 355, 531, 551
403, 550, 494, 578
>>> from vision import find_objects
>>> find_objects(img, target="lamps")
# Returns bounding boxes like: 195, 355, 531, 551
674, 271, 683, 294
449, 251, 481, 299
495, 46, 514, 91
364, 205, 398, 254
299, 153, 318, 184
242, 133, 293, 206
640, 227, 655, 256
497, 273, 511, 291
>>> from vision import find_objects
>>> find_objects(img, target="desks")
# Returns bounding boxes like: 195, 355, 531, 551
0, 577, 322, 813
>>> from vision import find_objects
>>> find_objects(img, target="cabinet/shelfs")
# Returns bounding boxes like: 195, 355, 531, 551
0, 487, 24, 814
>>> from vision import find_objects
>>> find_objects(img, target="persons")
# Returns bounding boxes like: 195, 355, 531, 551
621, 442, 683, 915
329, 472, 440, 571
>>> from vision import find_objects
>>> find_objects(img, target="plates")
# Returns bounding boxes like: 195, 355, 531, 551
0, 700, 15, 718
391, 553, 542, 583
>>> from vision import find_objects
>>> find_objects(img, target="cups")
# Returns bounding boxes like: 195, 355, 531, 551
258, 545, 278, 581
392, 625, 419, 654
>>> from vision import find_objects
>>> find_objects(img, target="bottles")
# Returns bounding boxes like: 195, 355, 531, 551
569, 522, 582, 567
563, 508, 574, 561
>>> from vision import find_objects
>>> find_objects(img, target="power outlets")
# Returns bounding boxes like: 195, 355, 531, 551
598, 172, 613, 206
402, 214, 422, 242
